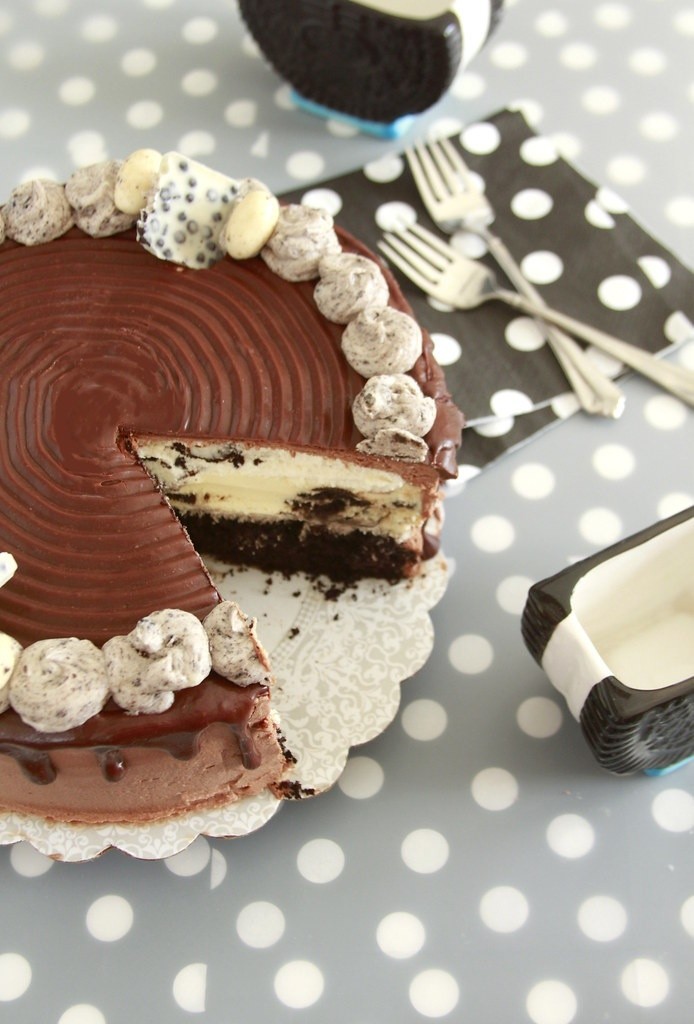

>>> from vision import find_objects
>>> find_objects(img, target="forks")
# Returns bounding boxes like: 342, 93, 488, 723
376, 222, 694, 432
401, 129, 626, 420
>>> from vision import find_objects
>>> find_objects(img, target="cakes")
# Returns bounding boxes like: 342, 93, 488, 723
0, 149, 466, 829
